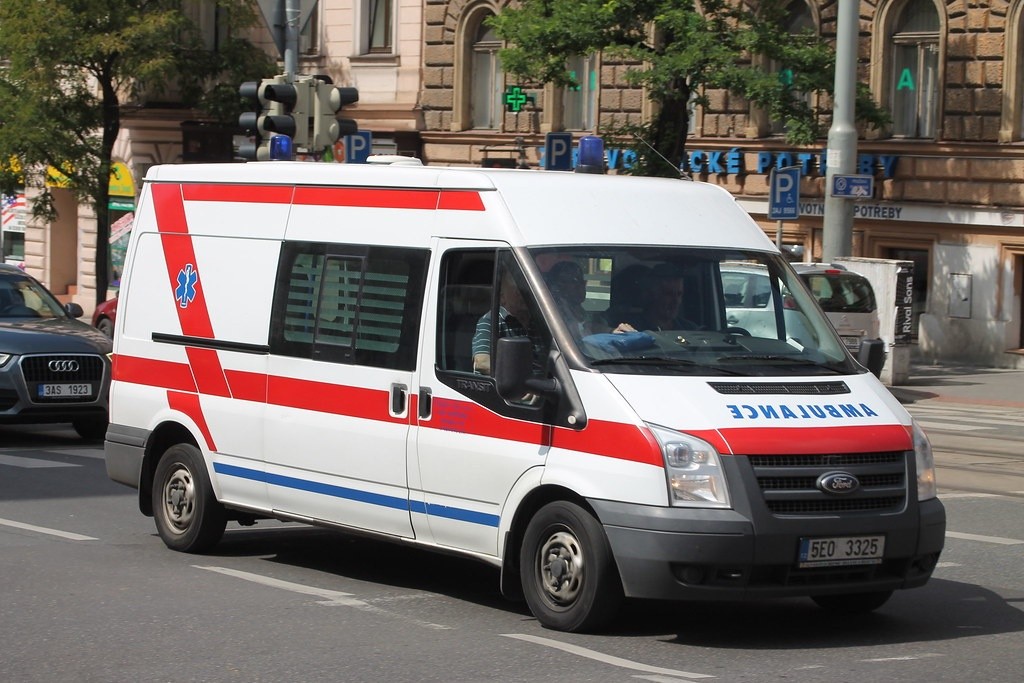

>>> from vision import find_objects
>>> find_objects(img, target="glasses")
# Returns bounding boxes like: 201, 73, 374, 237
563, 277, 588, 286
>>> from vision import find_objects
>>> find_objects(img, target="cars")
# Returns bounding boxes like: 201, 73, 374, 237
0, 263, 114, 439
91, 291, 120, 341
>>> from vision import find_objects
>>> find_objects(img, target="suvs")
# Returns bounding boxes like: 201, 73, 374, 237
715, 260, 886, 379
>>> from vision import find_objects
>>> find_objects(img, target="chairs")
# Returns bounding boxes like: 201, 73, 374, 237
452, 262, 500, 375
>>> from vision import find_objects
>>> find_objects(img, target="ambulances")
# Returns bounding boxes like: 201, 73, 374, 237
104, 134, 947, 632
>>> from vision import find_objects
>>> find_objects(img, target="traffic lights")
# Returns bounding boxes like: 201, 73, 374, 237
239, 78, 262, 162
263, 84, 310, 147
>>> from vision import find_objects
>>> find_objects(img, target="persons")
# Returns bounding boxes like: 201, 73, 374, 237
8, 291, 25, 306
626, 268, 699, 331
472, 262, 639, 405
